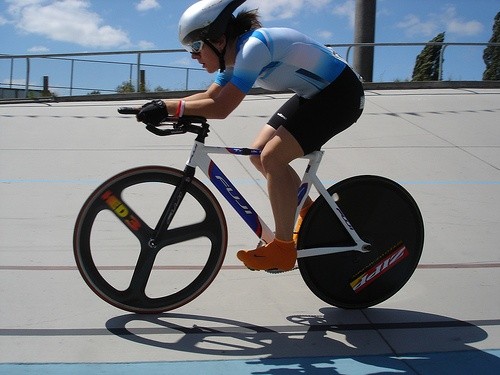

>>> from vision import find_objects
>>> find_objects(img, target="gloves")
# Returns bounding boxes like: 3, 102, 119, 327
136, 99, 168, 126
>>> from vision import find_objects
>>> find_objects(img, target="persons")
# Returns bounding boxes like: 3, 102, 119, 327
136, 0, 366, 271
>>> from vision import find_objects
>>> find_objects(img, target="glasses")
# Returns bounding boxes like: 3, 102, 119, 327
183, 39, 216, 53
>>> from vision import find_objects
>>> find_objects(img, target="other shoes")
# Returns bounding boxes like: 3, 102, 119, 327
292, 203, 314, 246
236, 240, 297, 272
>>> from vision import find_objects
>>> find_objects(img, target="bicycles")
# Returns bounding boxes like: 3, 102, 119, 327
72, 107, 425, 314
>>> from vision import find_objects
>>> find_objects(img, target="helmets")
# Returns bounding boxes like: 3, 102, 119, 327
178, 0, 246, 43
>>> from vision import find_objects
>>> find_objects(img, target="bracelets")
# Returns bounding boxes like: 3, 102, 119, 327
175, 100, 181, 117
179, 101, 185, 117
